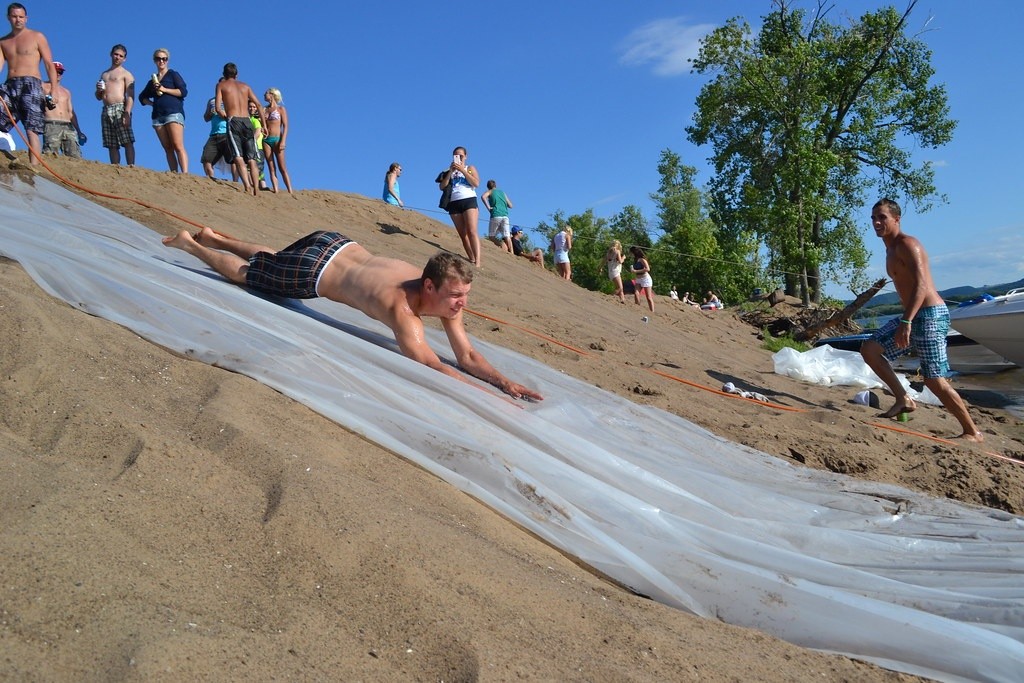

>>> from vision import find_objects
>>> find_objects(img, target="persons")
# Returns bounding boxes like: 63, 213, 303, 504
598, 239, 626, 300
383, 163, 403, 207
42, 62, 87, 157
670, 285, 723, 309
439, 147, 480, 266
162, 226, 543, 409
552, 226, 572, 280
200, 77, 239, 182
246, 89, 292, 193
138, 49, 188, 174
860, 198, 985, 442
481, 180, 513, 254
512, 226, 544, 268
215, 63, 269, 196
629, 246, 653, 312
0, 3, 58, 163
95, 44, 136, 165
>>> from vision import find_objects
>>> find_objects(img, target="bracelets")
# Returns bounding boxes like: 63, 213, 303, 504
900, 319, 912, 324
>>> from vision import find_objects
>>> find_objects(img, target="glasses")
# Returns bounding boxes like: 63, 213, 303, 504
57, 70, 64, 74
154, 56, 168, 62
519, 231, 523, 233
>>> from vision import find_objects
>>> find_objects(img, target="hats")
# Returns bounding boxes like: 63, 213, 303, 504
855, 389, 883, 410
512, 225, 521, 233
53, 61, 66, 71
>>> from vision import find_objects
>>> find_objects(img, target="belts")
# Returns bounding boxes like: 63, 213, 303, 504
45, 121, 71, 124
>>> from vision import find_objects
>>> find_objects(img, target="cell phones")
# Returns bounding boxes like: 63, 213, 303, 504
454, 155, 461, 165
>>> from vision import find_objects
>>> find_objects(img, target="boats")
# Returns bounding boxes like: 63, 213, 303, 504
813, 287, 1024, 377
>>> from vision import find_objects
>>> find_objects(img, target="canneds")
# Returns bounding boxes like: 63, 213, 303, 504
643, 316, 648, 322
99, 79, 105, 90
151, 73, 163, 96
896, 413, 907, 422
79, 136, 85, 146
